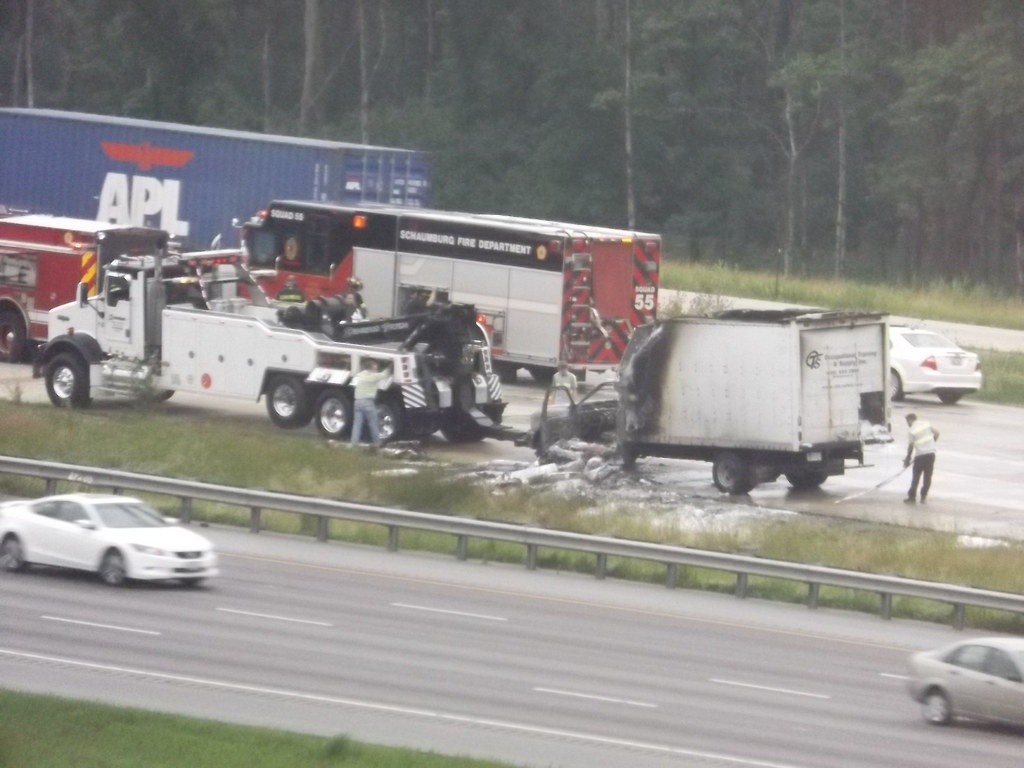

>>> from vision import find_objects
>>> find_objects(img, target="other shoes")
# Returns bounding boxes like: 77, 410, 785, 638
920, 500, 925, 504
904, 498, 915, 503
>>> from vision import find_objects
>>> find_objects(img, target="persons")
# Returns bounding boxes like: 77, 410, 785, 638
275, 274, 305, 303
550, 358, 578, 406
901, 412, 940, 507
343, 275, 369, 322
347, 358, 393, 454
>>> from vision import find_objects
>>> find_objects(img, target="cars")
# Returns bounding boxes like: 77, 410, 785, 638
884, 322, 983, 406
0, 492, 218, 589
904, 633, 1024, 730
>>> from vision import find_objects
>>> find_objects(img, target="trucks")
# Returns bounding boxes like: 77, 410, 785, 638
30, 247, 501, 452
0, 205, 170, 365
235, 198, 662, 379
530, 309, 892, 495
1, 107, 433, 257
